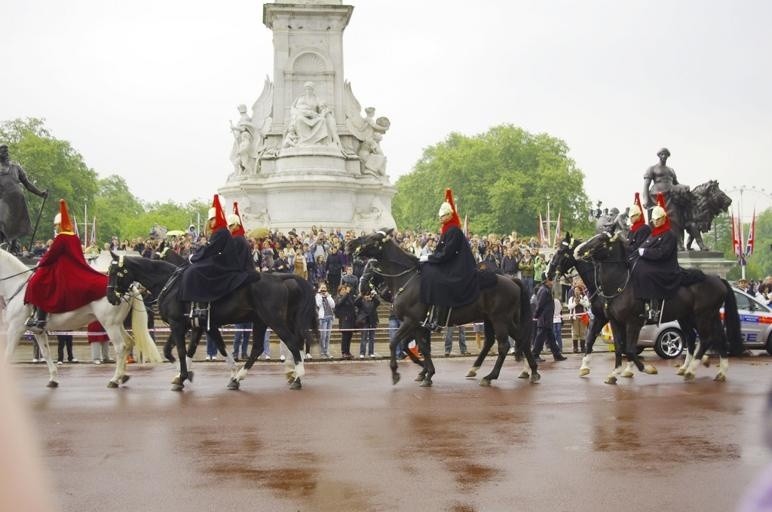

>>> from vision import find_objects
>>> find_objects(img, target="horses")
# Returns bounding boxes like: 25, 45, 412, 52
106, 248, 321, 392
544, 231, 711, 377
572, 223, 752, 385
150, 239, 306, 385
344, 228, 541, 387
0, 248, 163, 389
358, 257, 531, 381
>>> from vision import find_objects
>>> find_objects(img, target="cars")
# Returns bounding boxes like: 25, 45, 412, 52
634, 287, 771, 359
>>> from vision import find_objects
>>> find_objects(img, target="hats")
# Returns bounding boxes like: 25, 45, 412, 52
439, 202, 453, 217
208, 207, 216, 220
54, 213, 61, 224
652, 206, 666, 219
629, 204, 641, 216
228, 214, 240, 225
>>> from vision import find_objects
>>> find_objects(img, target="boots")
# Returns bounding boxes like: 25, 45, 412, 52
573, 340, 585, 352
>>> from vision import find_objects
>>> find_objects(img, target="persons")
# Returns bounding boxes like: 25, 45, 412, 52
589, 200, 631, 237
419, 202, 480, 309
27, 235, 78, 363
643, 148, 685, 250
616, 204, 650, 270
24, 212, 109, 329
0, 143, 45, 254
177, 207, 256, 300
108, 227, 594, 362
734, 272, 772, 311
627, 206, 682, 324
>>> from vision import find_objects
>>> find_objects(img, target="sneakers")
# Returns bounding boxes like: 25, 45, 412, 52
205, 354, 286, 361
93, 356, 114, 364
303, 353, 375, 359
33, 357, 77, 365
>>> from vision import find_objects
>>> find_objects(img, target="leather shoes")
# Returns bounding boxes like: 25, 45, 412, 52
534, 356, 546, 361
639, 311, 657, 322
184, 310, 206, 320
420, 321, 441, 332
555, 355, 567, 360
28, 320, 45, 329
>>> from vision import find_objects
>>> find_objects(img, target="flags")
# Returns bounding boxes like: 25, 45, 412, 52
730, 201, 755, 267
74, 205, 97, 251
